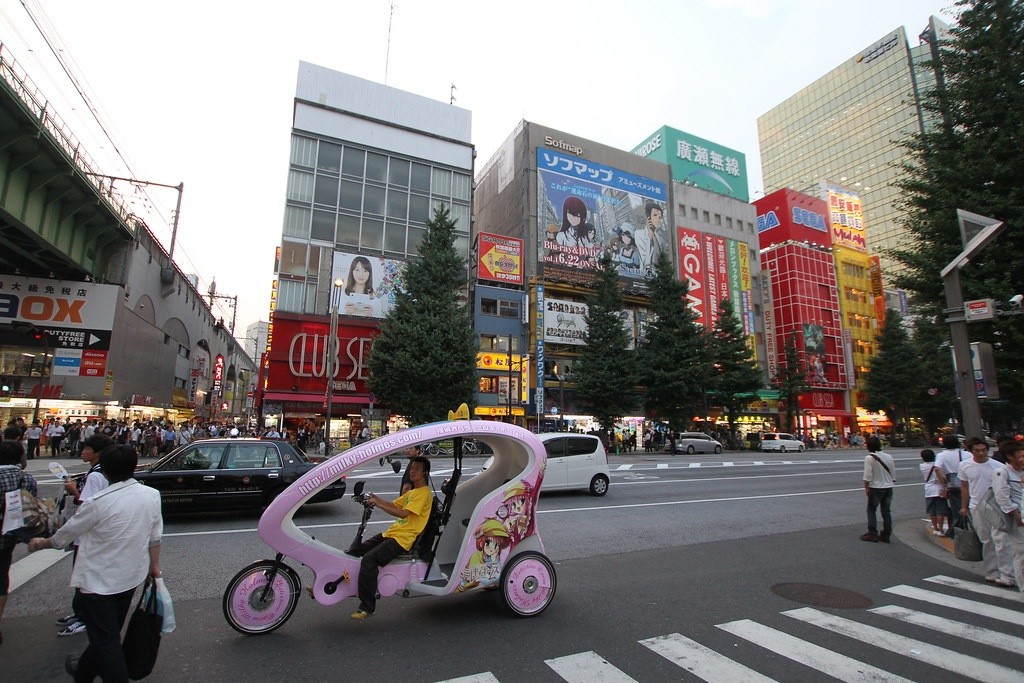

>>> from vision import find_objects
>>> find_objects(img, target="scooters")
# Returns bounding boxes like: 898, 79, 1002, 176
223, 405, 557, 636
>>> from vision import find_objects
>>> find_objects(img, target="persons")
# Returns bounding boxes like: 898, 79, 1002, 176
354, 425, 389, 445
339, 256, 382, 317
546, 328, 586, 338
577, 427, 676, 456
257, 423, 323, 454
710, 429, 742, 451
400, 446, 429, 497
0, 415, 257, 469
306, 456, 433, 619
27, 445, 163, 683
547, 301, 585, 314
793, 430, 904, 448
55, 434, 113, 635
549, 361, 570, 378
919, 431, 1024, 593
859, 436, 895, 542
0, 441, 37, 644
814, 357, 827, 382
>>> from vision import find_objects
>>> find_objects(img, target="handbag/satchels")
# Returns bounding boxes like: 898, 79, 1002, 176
945, 473, 961, 489
0, 470, 62, 544
142, 570, 177, 634
55, 482, 81, 553
121, 575, 165, 681
976, 468, 1014, 534
430, 495, 444, 519
952, 513, 983, 562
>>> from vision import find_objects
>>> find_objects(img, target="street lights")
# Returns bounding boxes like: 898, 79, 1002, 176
324, 278, 345, 456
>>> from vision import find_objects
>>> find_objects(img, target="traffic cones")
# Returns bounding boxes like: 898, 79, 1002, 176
615, 446, 620, 456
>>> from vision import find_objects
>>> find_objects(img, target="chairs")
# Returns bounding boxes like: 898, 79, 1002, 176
208, 451, 222, 468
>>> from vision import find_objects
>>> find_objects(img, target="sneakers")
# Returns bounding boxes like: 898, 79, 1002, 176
56, 621, 87, 636
350, 609, 376, 619
55, 613, 79, 626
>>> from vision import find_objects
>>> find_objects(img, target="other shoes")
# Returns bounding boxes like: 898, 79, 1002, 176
859, 532, 879, 543
65, 653, 95, 683
995, 578, 1014, 587
984, 570, 1000, 582
877, 530, 891, 543
933, 528, 947, 537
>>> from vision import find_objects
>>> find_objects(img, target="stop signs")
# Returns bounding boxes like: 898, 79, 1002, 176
248, 392, 254, 397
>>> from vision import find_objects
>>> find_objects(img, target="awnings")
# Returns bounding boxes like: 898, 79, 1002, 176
808, 409, 859, 417
264, 392, 380, 404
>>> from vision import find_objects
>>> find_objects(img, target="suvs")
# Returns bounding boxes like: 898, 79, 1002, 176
761, 433, 805, 453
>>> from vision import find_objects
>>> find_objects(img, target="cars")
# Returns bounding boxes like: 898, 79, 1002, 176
133, 438, 347, 526
937, 430, 997, 447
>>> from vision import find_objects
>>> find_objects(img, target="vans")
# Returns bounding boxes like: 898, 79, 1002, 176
479, 433, 611, 497
663, 432, 723, 455
